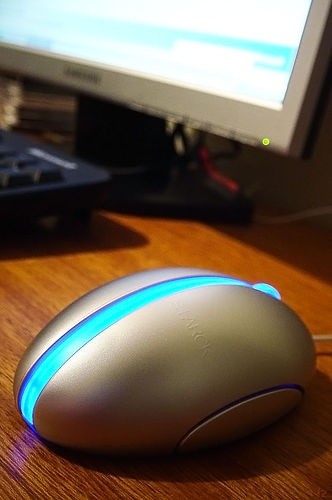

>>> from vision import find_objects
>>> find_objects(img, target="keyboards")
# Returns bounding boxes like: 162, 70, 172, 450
0, 125, 111, 213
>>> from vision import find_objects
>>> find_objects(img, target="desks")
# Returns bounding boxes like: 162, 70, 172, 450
0, 105, 331, 499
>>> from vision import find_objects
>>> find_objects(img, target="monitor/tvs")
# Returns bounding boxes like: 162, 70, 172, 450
0, 0, 331, 186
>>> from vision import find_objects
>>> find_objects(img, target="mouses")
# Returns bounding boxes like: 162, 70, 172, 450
11, 265, 316, 451
